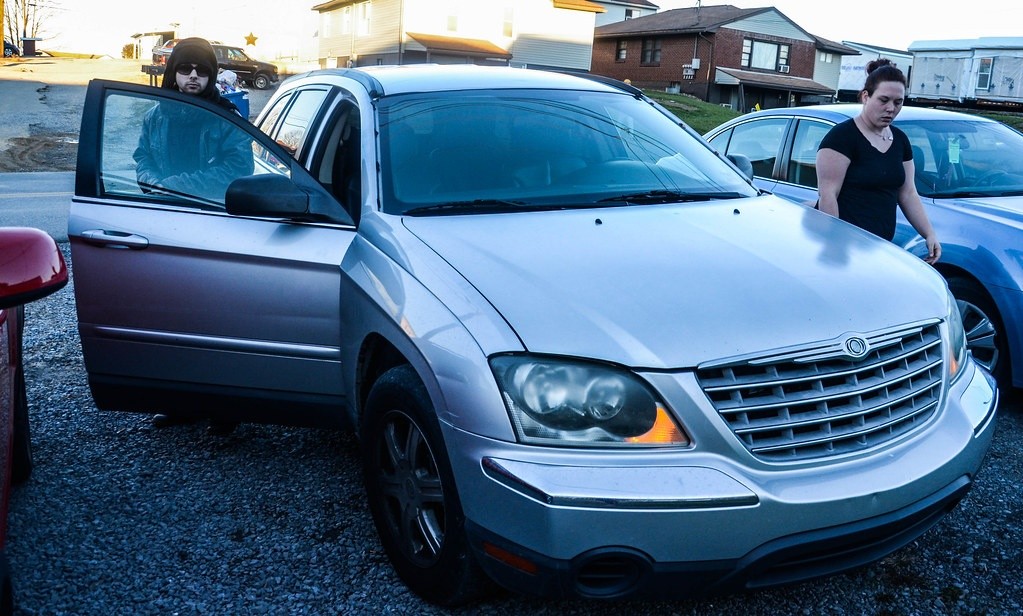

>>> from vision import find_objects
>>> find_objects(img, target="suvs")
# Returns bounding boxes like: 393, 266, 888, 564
157, 40, 280, 91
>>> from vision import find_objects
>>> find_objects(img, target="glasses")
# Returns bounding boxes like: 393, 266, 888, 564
176, 63, 212, 77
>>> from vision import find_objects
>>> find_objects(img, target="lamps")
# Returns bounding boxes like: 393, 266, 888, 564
952, 84, 956, 88
1009, 84, 1014, 88
990, 84, 996, 88
921, 83, 925, 87
936, 84, 940, 87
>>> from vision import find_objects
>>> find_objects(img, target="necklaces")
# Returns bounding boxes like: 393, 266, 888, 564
860, 114, 893, 141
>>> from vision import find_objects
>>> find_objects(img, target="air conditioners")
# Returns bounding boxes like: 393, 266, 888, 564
779, 65, 789, 73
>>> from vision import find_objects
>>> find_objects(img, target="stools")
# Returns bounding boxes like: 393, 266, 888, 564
751, 108, 755, 112
720, 104, 732, 109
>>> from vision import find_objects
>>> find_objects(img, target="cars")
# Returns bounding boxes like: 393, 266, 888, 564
649, 102, 1022, 411
2, 40, 21, 59
68, 46, 1008, 612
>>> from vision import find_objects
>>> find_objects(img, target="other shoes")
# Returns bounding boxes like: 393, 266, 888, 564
207, 420, 238, 435
152, 413, 191, 426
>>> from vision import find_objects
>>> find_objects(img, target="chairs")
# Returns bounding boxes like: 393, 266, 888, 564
911, 145, 940, 193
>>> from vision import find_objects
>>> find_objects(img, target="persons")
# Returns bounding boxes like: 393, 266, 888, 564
618, 79, 634, 138
814, 58, 942, 264
132, 37, 255, 433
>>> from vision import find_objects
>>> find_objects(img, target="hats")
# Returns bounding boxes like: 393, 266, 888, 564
171, 46, 214, 79
